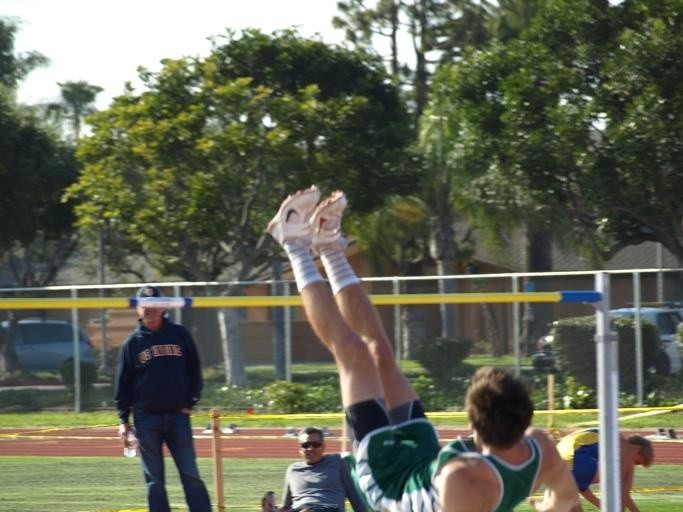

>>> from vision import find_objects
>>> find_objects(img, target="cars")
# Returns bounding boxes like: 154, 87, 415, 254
0, 317, 96, 371
532, 301, 683, 374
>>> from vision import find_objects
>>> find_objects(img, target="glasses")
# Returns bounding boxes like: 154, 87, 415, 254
301, 442, 321, 448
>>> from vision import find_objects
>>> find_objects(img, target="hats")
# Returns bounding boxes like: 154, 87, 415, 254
135, 285, 164, 298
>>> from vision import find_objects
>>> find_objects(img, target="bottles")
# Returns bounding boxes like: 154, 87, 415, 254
124, 427, 136, 458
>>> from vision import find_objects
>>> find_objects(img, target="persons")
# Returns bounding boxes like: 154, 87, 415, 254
265, 185, 582, 512
113, 286, 210, 512
554, 428, 652, 512
262, 427, 368, 512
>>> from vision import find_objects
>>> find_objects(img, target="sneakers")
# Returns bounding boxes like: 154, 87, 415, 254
308, 189, 348, 257
266, 183, 322, 247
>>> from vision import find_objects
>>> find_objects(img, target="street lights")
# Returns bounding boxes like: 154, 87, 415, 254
92, 193, 118, 370
